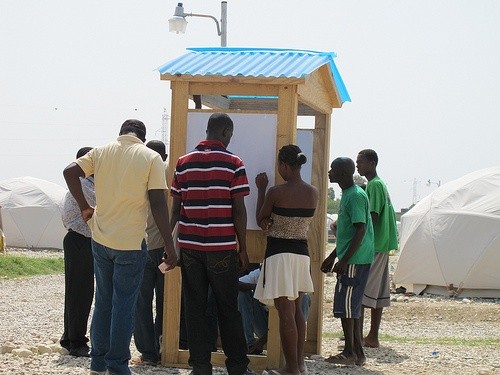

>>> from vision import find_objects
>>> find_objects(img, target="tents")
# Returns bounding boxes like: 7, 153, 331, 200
395, 166, 500, 298
0, 176, 68, 250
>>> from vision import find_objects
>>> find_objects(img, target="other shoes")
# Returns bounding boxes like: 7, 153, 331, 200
142, 359, 157, 365
69, 347, 91, 357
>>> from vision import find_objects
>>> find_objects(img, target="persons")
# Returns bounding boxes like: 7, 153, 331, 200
170, 112, 256, 375
330, 149, 399, 348
255, 144, 319, 375
64, 120, 177, 375
319, 157, 375, 365
133, 139, 169, 366
59, 147, 96, 357
238, 263, 310, 354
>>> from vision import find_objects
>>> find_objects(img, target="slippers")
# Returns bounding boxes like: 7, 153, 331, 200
263, 370, 302, 375
323, 354, 355, 365
362, 338, 376, 348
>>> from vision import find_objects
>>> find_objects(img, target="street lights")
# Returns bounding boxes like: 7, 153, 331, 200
166, 0, 230, 47
